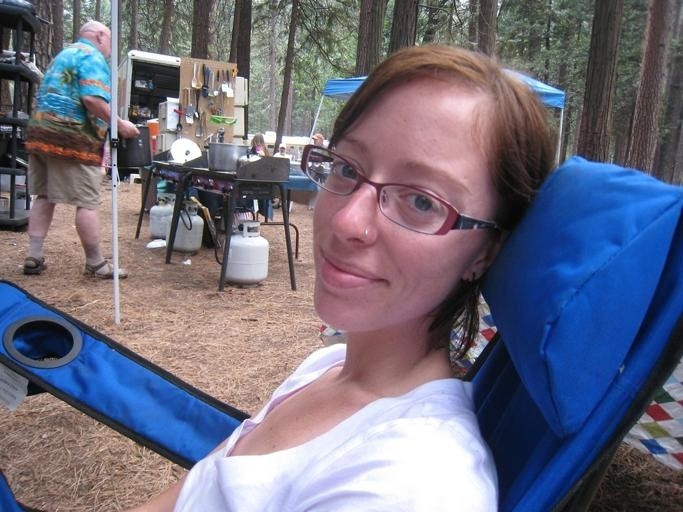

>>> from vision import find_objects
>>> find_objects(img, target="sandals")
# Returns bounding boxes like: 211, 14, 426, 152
23, 257, 47, 274
86, 260, 128, 279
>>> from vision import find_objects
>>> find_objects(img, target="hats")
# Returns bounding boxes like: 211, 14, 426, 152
280, 145, 286, 149
312, 134, 323, 140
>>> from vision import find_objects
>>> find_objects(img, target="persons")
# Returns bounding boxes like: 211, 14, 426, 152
249, 134, 274, 223
271, 144, 290, 208
116, 43, 558, 512
308, 133, 332, 211
22, 19, 140, 280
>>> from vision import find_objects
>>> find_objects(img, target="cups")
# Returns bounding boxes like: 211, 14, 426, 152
0, 197, 8, 214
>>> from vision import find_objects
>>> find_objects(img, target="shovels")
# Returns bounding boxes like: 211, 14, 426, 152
192, 64, 197, 87
196, 109, 203, 137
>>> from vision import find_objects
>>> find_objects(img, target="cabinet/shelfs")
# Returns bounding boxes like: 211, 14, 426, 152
0, 0, 44, 232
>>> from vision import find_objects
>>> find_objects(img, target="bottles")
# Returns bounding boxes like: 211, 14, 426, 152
147, 80, 153, 90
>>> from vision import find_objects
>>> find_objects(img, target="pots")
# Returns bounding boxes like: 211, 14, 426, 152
107, 124, 151, 168
203, 142, 249, 172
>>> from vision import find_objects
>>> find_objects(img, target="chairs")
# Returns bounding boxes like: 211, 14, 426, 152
0, 155, 681, 512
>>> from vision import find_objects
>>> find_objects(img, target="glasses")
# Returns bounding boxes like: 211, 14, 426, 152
300, 145, 501, 235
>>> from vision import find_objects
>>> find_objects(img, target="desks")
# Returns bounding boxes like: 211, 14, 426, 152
130, 161, 297, 294
